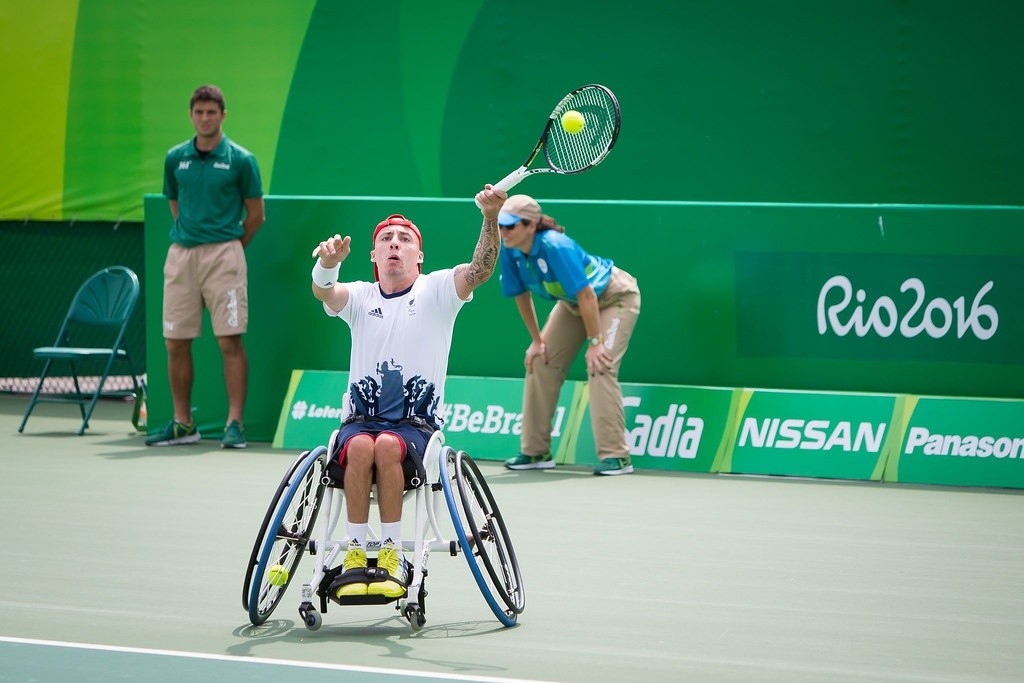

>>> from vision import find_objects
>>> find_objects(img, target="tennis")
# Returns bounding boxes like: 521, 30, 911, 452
561, 110, 586, 133
267, 565, 287, 587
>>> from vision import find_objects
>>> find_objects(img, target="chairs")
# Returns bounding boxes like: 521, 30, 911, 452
17, 265, 144, 436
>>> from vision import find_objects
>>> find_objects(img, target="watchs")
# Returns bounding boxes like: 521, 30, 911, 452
588, 337, 604, 345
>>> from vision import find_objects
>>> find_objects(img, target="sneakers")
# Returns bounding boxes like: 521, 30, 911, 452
145, 416, 201, 446
367, 543, 409, 597
220, 418, 247, 449
593, 457, 634, 476
503, 452, 555, 470
334, 543, 367, 598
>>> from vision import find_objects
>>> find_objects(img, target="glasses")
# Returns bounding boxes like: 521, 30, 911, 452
498, 224, 522, 230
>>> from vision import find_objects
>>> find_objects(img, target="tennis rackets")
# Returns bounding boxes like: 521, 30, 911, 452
475, 83, 622, 210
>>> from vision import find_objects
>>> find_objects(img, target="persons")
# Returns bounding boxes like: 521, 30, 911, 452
498, 194, 640, 476
312, 183, 507, 599
145, 83, 263, 448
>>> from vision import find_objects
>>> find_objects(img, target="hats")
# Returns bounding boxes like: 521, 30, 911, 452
496, 194, 541, 225
373, 214, 422, 281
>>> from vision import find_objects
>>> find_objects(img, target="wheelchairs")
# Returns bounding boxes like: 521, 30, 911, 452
241, 429, 524, 633
